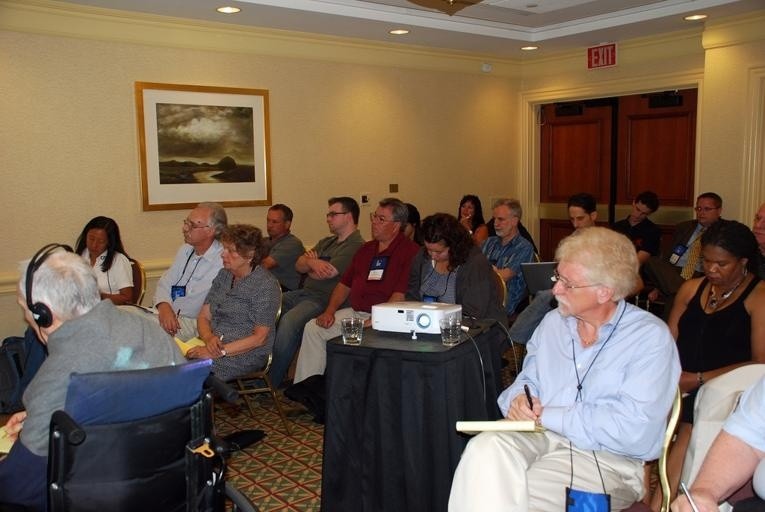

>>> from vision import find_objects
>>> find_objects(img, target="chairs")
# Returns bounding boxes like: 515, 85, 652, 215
618, 384, 681, 512
491, 264, 507, 309
124, 258, 146, 306
207, 279, 294, 435
532, 251, 540, 263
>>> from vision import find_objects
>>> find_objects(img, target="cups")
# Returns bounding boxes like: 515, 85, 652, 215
341, 317, 364, 346
439, 319, 461, 346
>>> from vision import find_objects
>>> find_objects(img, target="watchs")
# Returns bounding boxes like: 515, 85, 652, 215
221, 350, 227, 357
695, 370, 704, 385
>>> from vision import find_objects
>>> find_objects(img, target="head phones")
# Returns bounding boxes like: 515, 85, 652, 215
25, 243, 73, 329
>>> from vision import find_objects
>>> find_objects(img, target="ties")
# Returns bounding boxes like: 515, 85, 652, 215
679, 230, 711, 278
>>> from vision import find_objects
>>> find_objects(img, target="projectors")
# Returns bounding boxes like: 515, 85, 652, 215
371, 301, 462, 341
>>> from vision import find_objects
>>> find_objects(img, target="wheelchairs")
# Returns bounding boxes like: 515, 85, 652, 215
46, 359, 263, 512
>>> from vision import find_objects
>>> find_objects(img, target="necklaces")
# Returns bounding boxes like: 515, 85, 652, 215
709, 268, 749, 309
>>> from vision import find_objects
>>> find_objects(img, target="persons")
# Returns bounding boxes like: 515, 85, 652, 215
406, 213, 507, 332
288, 198, 420, 420
458, 194, 488, 251
403, 202, 419, 246
607, 192, 664, 303
183, 224, 281, 409
0, 243, 189, 511
75, 216, 133, 306
256, 203, 306, 293
644, 219, 765, 511
153, 203, 226, 342
505, 192, 598, 364
448, 229, 682, 511
751, 205, 763, 260
265, 196, 364, 394
481, 197, 535, 318
639, 192, 723, 322
670, 372, 765, 512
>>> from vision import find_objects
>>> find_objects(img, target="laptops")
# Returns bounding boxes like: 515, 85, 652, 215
519, 261, 563, 296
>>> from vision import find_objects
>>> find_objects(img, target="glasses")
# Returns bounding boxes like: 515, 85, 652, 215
694, 204, 720, 213
369, 214, 405, 223
327, 210, 354, 219
549, 271, 607, 289
181, 219, 211, 230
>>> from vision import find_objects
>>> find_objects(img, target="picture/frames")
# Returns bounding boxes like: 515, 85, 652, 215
134, 81, 272, 212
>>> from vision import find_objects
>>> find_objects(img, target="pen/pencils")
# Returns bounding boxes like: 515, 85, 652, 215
523, 384, 536, 411
176, 308, 180, 316
218, 332, 224, 339
682, 482, 700, 512
